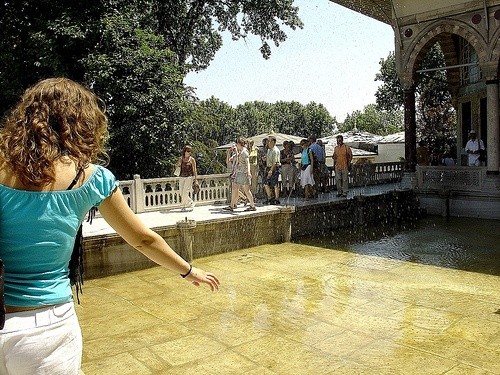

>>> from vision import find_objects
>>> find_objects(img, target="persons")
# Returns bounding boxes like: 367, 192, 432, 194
176, 146, 197, 209
222, 134, 354, 211
1, 77, 221, 375
465, 131, 485, 167
415, 137, 457, 167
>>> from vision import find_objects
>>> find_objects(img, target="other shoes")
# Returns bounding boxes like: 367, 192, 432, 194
233, 204, 237, 209
341, 195, 347, 198
336, 194, 342, 197
262, 197, 274, 204
244, 198, 248, 206
181, 208, 186, 212
246, 206, 256, 211
189, 202, 195, 211
221, 206, 233, 213
268, 199, 280, 205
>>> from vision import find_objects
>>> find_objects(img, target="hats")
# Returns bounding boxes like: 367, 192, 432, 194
469, 129, 476, 134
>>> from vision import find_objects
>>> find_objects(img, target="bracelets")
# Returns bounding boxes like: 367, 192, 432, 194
179, 264, 192, 278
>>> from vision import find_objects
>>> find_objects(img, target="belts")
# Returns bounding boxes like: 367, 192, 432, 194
5, 304, 56, 313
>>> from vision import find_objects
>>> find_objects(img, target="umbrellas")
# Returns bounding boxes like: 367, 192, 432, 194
216, 128, 407, 163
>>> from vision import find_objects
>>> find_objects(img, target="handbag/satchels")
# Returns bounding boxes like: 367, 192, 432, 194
299, 163, 307, 171
174, 166, 181, 177
